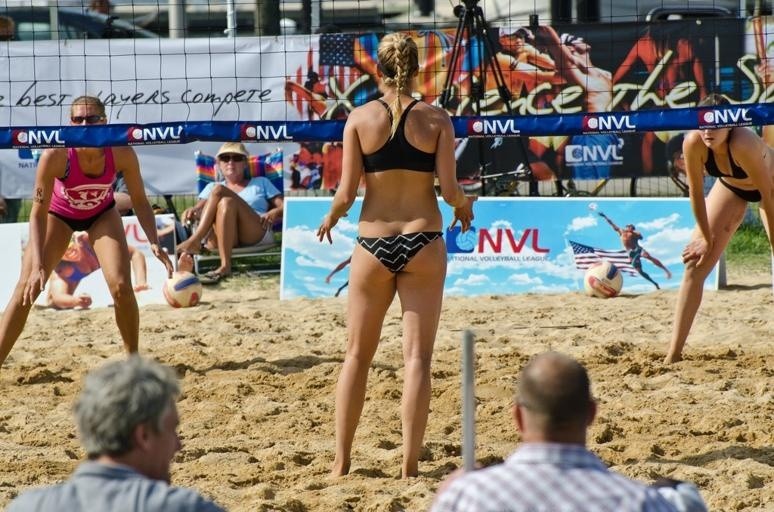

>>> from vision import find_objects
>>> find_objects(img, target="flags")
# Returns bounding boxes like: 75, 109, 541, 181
571, 239, 640, 276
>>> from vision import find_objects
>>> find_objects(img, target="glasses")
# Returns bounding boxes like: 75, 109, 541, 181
70, 115, 104, 123
219, 155, 243, 162
509, 32, 526, 39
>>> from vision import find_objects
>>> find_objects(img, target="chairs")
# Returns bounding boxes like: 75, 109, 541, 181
194, 149, 285, 280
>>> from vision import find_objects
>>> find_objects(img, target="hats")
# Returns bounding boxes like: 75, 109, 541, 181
215, 142, 249, 159
499, 24, 530, 38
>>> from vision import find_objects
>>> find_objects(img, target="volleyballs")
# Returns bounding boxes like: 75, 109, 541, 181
583, 259, 623, 299
162, 270, 203, 308
587, 202, 598, 212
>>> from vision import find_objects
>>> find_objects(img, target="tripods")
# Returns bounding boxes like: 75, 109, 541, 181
438, 0, 539, 198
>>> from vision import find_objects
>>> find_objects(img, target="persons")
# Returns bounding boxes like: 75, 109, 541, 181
48, 232, 154, 310
613, 22, 707, 175
532, 26, 613, 182
661, 95, 774, 367
484, 26, 566, 180
316, 32, 478, 479
525, 85, 570, 169
430, 349, 707, 512
0, 97, 175, 357
176, 140, 283, 281
597, 210, 671, 291
0, 354, 223, 512
